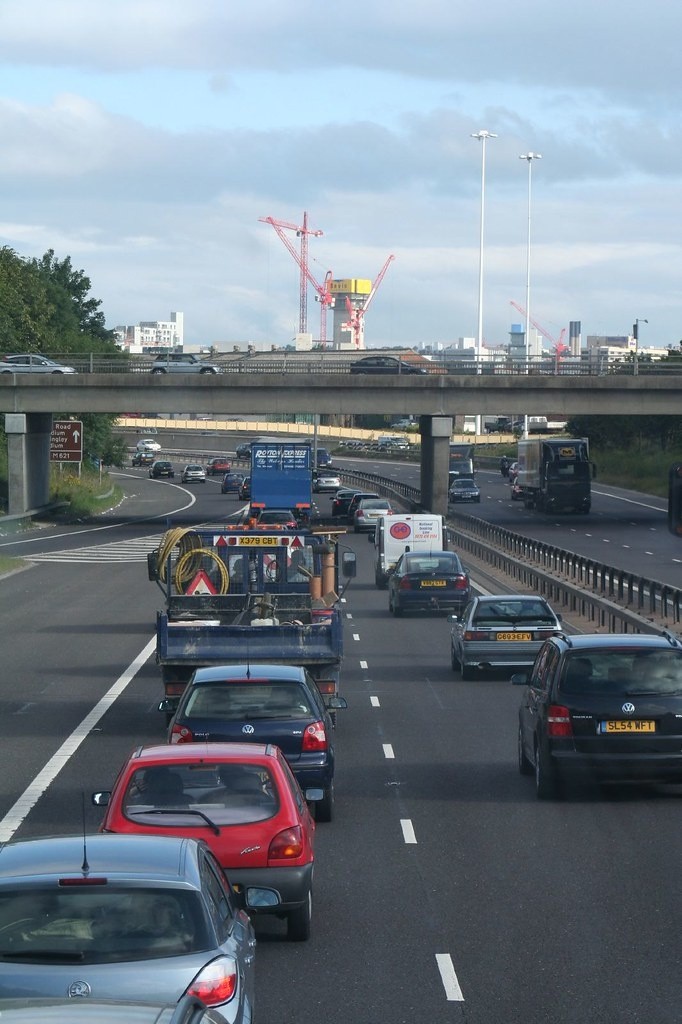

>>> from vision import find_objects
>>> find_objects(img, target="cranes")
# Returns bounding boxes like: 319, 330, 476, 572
510, 299, 571, 375
257, 210, 396, 352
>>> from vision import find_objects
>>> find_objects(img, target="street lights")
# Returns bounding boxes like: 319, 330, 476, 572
517, 152, 545, 376
470, 130, 499, 375
633, 318, 649, 377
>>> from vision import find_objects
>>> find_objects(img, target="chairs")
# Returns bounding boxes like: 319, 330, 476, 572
141, 772, 195, 803
626, 654, 668, 697
408, 561, 423, 574
563, 658, 595, 695
227, 774, 270, 804
437, 558, 453, 572
264, 686, 302, 716
201, 691, 232, 716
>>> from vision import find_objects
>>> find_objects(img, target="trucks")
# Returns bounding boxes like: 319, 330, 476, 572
368, 513, 448, 589
378, 436, 410, 450
449, 442, 476, 485
484, 416, 570, 433
518, 438, 597, 516
146, 521, 358, 724
249, 442, 313, 519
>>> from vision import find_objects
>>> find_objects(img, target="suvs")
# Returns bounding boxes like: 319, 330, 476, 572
511, 630, 682, 800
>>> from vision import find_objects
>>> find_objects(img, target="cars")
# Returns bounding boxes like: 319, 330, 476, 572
338, 440, 388, 453
0, 355, 79, 375
131, 439, 252, 501
156, 663, 348, 821
0, 830, 254, 1024
447, 595, 564, 680
150, 353, 226, 374
448, 461, 524, 503
392, 418, 412, 431
348, 356, 432, 376
91, 741, 314, 943
257, 508, 299, 529
329, 489, 395, 533
310, 448, 341, 491
386, 551, 472, 618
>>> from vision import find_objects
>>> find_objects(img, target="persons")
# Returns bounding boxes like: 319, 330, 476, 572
197, 765, 245, 805
613, 654, 676, 695
205, 688, 231, 713
129, 769, 191, 806
270, 688, 298, 709
499, 456, 510, 474
